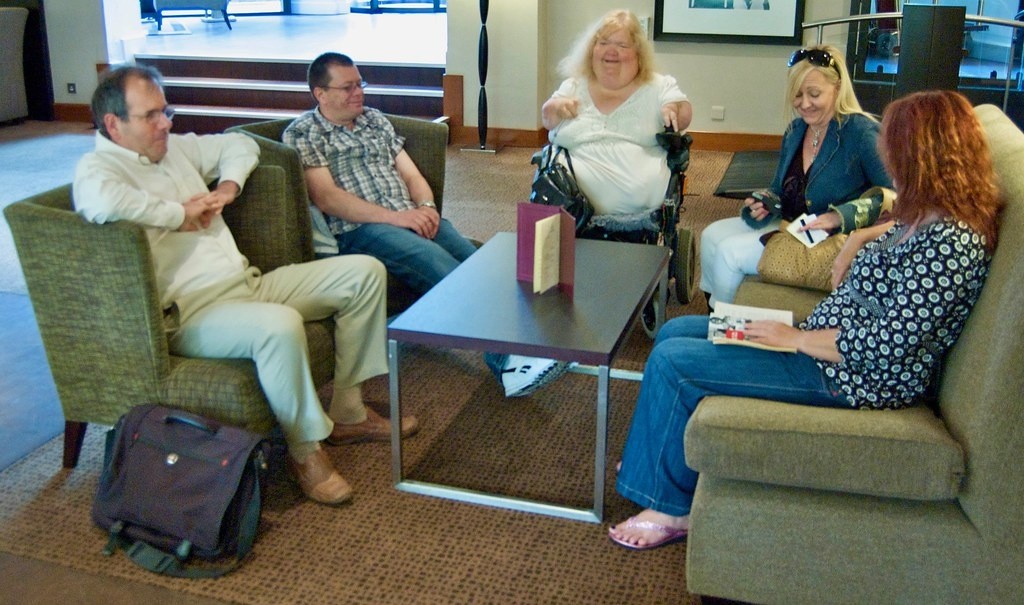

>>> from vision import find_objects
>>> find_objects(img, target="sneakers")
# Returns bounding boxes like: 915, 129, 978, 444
501, 353, 577, 398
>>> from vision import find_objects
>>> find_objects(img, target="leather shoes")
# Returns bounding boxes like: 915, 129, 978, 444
327, 405, 419, 442
284, 444, 352, 507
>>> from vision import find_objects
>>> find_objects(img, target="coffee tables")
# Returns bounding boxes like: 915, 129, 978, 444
382, 232, 675, 530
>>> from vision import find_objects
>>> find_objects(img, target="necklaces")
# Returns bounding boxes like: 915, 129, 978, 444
807, 120, 830, 160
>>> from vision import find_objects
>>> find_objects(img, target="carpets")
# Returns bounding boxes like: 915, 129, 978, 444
5, 130, 743, 603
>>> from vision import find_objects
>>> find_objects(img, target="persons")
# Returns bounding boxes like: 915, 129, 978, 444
281, 50, 572, 398
606, 90, 1007, 549
542, 9, 696, 340
697, 41, 898, 315
71, 62, 419, 507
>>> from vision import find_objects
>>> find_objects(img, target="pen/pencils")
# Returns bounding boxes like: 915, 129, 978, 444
800, 219, 814, 244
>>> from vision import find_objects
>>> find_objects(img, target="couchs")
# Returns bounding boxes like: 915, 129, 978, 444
681, 97, 1024, 605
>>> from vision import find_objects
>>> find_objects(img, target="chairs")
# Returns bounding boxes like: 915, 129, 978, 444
1, 161, 346, 473
221, 111, 453, 320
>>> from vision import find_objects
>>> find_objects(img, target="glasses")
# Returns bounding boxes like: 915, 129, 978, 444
318, 81, 369, 95
121, 106, 177, 126
786, 48, 842, 82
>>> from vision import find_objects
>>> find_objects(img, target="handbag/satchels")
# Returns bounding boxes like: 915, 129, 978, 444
91, 402, 275, 577
530, 143, 593, 237
757, 218, 858, 293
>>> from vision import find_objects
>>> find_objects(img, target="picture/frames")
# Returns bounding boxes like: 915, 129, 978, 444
652, 0, 806, 48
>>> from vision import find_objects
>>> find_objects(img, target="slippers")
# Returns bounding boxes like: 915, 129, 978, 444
608, 508, 696, 551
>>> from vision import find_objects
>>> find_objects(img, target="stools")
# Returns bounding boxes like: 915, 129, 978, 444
533, 122, 697, 305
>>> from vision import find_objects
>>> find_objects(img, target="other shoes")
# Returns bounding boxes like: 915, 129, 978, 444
640, 298, 659, 340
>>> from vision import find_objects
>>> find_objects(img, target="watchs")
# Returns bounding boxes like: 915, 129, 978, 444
418, 200, 437, 208
